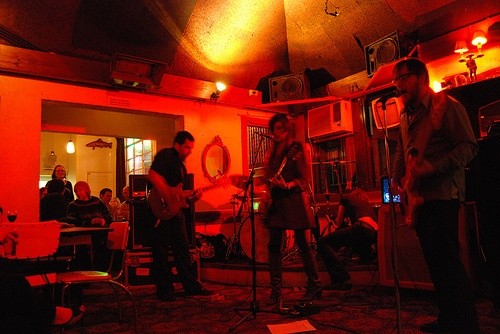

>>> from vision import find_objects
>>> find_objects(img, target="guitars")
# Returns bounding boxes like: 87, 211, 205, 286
397, 92, 448, 227
147, 177, 229, 221
258, 141, 304, 214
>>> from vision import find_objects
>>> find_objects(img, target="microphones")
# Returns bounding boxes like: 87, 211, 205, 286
254, 131, 274, 140
377, 89, 401, 102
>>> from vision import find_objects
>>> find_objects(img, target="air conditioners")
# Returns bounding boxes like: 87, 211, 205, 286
306, 99, 353, 140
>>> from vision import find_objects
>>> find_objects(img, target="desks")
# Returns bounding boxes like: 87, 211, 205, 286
60, 227, 114, 271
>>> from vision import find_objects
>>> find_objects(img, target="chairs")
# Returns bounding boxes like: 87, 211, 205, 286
58, 222, 140, 334
0, 219, 68, 334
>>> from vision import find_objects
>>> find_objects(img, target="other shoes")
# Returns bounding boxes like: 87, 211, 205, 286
323, 279, 353, 291
160, 293, 176, 302
269, 295, 287, 309
185, 283, 211, 296
288, 301, 321, 318
417, 314, 480, 334
351, 248, 377, 265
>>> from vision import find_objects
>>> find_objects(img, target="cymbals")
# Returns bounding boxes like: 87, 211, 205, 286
229, 174, 255, 191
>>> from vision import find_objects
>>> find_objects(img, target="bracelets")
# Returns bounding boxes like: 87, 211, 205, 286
288, 183, 291, 190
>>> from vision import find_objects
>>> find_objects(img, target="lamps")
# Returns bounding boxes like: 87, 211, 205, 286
66, 134, 76, 154
453, 29, 488, 82
210, 82, 227, 98
49, 133, 57, 165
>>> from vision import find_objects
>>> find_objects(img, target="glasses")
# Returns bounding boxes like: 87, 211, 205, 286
391, 72, 414, 86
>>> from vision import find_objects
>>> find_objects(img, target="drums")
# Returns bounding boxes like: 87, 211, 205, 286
239, 211, 296, 263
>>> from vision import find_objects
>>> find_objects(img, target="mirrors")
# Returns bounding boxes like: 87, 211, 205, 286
201, 134, 231, 182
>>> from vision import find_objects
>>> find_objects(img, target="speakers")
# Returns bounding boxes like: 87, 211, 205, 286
129, 198, 158, 248
364, 28, 404, 78
265, 72, 305, 102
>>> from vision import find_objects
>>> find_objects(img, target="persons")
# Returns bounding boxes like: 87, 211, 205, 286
389, 58, 481, 334
316, 186, 378, 290
40, 165, 130, 271
0, 261, 89, 334
148, 130, 214, 301
254, 112, 323, 305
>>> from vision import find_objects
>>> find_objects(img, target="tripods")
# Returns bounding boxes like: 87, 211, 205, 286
226, 136, 297, 333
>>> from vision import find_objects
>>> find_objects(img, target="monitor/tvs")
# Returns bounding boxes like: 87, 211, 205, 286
381, 175, 401, 203
